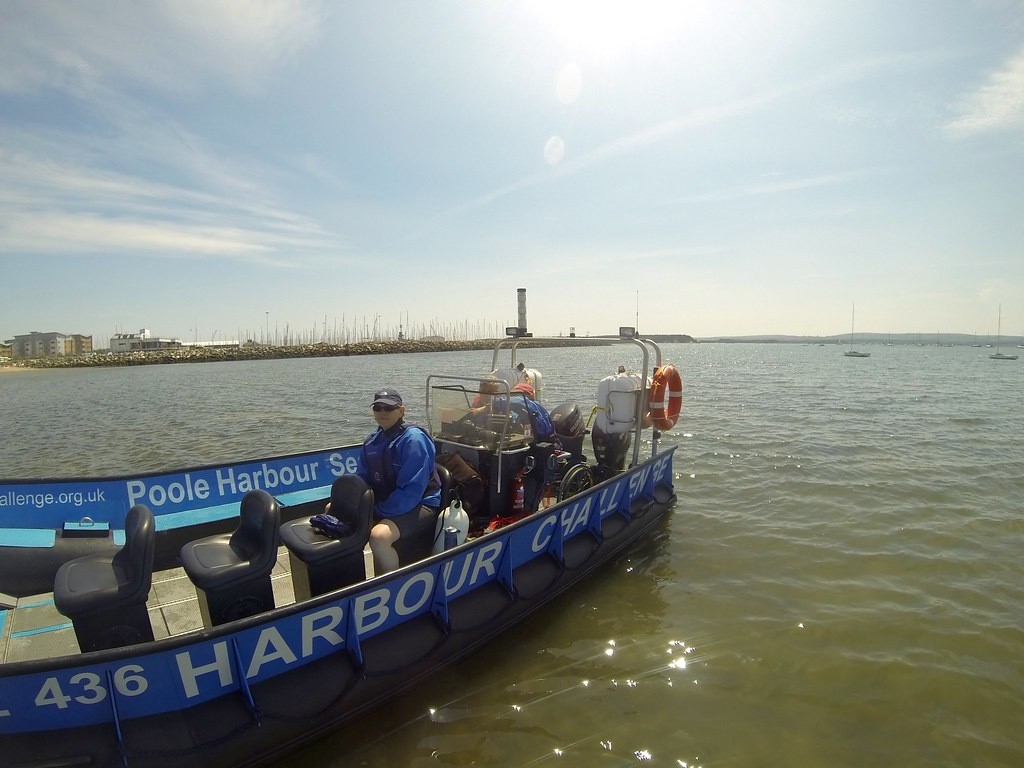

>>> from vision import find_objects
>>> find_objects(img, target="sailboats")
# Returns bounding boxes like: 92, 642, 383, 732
988, 304, 1019, 360
843, 302, 872, 357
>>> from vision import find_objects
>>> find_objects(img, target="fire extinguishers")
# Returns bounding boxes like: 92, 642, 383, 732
511, 471, 524, 513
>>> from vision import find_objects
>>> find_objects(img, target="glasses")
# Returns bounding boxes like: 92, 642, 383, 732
373, 405, 404, 416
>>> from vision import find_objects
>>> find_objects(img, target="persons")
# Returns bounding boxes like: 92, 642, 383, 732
326, 388, 442, 579
468, 374, 555, 513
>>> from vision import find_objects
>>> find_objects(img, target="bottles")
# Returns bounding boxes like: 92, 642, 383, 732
444, 527, 460, 550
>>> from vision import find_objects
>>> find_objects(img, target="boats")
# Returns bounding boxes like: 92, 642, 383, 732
986, 344, 993, 348
1, 327, 685, 768
1018, 345, 1024, 348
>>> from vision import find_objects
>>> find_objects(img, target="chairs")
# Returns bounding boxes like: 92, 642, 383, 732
52, 504, 157, 654
280, 475, 373, 601
394, 462, 452, 569
180, 489, 280, 629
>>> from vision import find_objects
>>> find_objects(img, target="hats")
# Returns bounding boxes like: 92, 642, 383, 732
512, 383, 535, 399
369, 387, 402, 407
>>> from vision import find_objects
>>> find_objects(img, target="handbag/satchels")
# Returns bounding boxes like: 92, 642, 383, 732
309, 514, 350, 537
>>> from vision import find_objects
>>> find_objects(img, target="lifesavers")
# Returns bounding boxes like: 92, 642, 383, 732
649, 363, 684, 432
